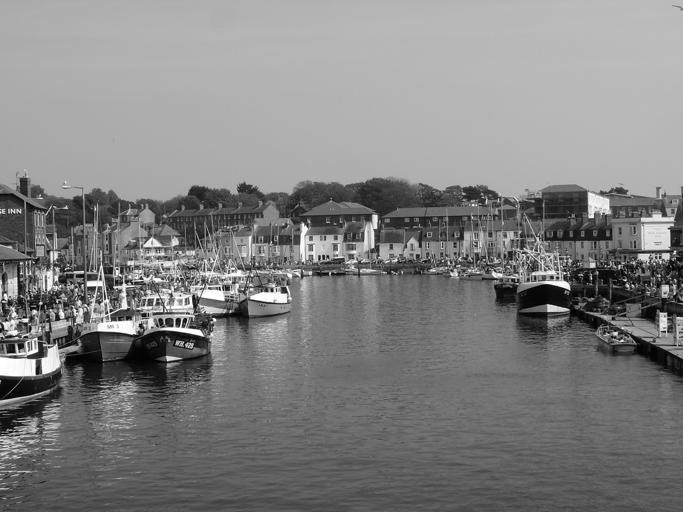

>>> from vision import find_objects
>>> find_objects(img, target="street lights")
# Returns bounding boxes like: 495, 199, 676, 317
60, 182, 88, 308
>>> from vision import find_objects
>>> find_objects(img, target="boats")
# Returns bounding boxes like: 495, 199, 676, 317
594, 324, 637, 353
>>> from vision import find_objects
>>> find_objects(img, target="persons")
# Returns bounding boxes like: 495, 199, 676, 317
1, 282, 84, 325
603, 329, 635, 343
